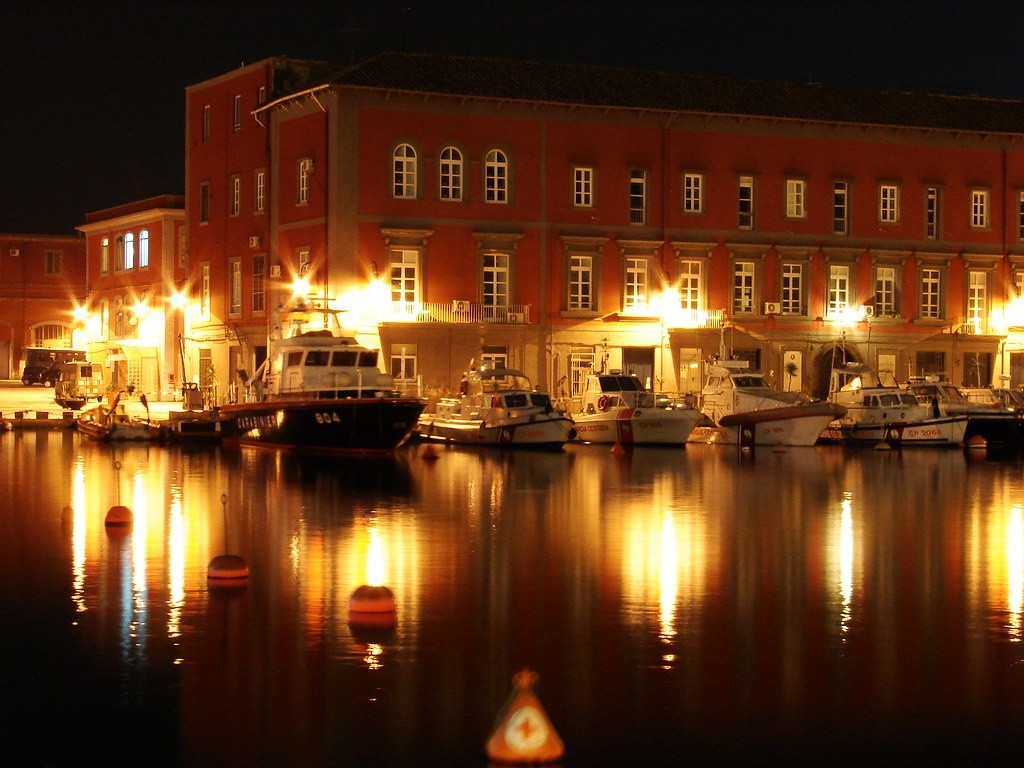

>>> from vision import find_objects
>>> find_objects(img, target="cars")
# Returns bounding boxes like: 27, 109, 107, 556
22, 365, 58, 388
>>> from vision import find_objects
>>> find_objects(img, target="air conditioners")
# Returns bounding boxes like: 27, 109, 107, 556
507, 313, 523, 323
270, 265, 280, 277
860, 305, 873, 316
250, 237, 259, 248
453, 300, 470, 312
10, 249, 20, 256
765, 302, 780, 315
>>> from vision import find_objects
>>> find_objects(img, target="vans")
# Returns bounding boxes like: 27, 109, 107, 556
54, 361, 107, 402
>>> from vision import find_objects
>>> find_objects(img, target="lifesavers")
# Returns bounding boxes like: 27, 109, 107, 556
597, 396, 609, 411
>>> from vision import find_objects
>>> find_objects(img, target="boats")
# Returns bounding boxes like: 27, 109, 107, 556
76, 385, 161, 441
164, 319, 1024, 457
